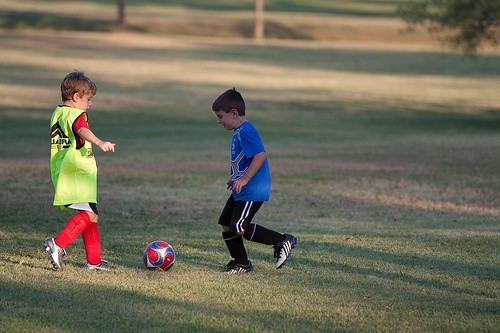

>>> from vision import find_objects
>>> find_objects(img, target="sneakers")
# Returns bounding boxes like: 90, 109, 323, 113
44, 237, 66, 271
224, 259, 254, 275
85, 259, 117, 271
272, 233, 297, 269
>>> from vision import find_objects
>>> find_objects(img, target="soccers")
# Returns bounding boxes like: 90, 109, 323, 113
143, 240, 176, 272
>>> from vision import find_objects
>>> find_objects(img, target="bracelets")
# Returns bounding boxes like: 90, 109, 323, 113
244, 173, 251, 180
242, 174, 249, 182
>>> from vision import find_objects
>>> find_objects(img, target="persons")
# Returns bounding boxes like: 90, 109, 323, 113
43, 68, 119, 274
212, 87, 297, 275
